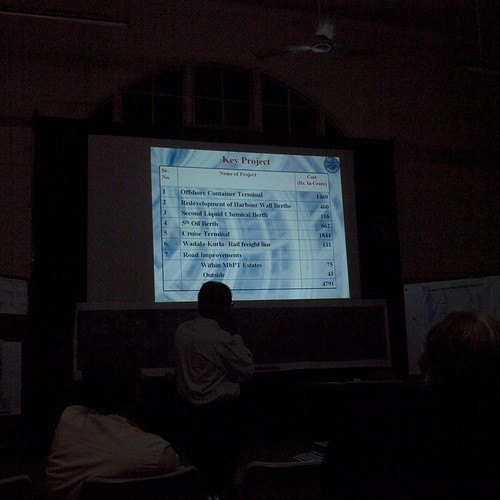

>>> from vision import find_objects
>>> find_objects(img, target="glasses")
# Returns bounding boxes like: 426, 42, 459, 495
221, 301, 234, 307
120, 374, 145, 387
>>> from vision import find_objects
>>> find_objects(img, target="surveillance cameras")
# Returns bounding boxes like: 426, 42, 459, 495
311, 21, 336, 54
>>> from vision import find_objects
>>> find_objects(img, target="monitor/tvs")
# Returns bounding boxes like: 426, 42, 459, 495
0, 275, 30, 318
402, 273, 500, 377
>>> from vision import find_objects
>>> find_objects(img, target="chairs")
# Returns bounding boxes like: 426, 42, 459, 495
0, 457, 327, 500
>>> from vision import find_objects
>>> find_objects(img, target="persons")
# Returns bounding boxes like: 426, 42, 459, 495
172, 282, 254, 500
418, 309, 500, 397
44, 358, 179, 500
